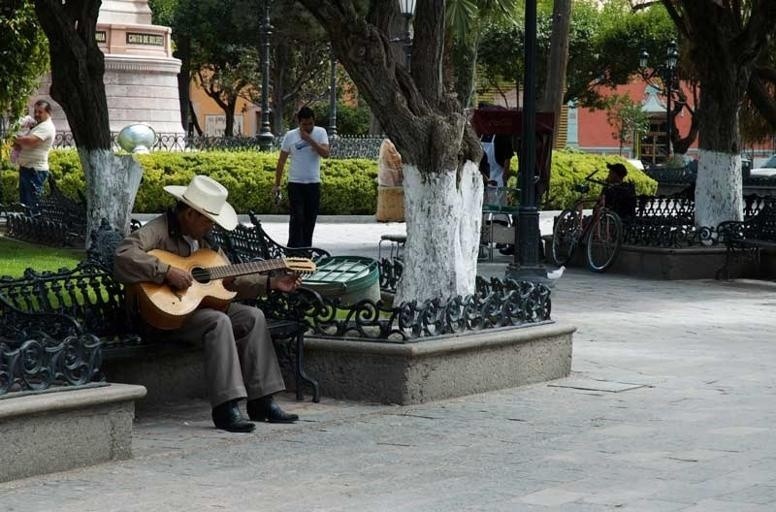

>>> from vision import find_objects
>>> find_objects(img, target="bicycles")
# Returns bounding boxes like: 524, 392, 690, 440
551, 169, 632, 272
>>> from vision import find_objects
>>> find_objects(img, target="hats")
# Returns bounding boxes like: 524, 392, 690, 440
162, 175, 238, 231
607, 163, 626, 176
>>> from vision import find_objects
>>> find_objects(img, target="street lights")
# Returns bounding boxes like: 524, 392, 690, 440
638, 38, 680, 183
388, 0, 418, 75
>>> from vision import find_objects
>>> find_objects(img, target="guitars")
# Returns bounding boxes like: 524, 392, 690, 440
133, 249, 316, 329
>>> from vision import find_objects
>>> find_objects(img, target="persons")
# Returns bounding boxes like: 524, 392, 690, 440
479, 104, 514, 205
273, 107, 331, 255
12, 99, 55, 211
596, 162, 631, 217
113, 175, 303, 433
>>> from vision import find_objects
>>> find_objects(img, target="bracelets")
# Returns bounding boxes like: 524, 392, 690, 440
307, 139, 313, 143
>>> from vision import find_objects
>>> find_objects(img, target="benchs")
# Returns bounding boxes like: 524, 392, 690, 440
213, 212, 329, 272
0, 217, 327, 435
593, 182, 775, 226
11, 171, 144, 239
712, 209, 776, 286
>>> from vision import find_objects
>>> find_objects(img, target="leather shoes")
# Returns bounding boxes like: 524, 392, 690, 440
212, 403, 255, 432
495, 242, 512, 255
246, 397, 298, 422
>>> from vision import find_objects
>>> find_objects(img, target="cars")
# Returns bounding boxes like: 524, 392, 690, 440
748, 154, 776, 182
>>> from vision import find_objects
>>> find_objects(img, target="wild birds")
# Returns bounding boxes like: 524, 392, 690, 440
547, 265, 566, 280
